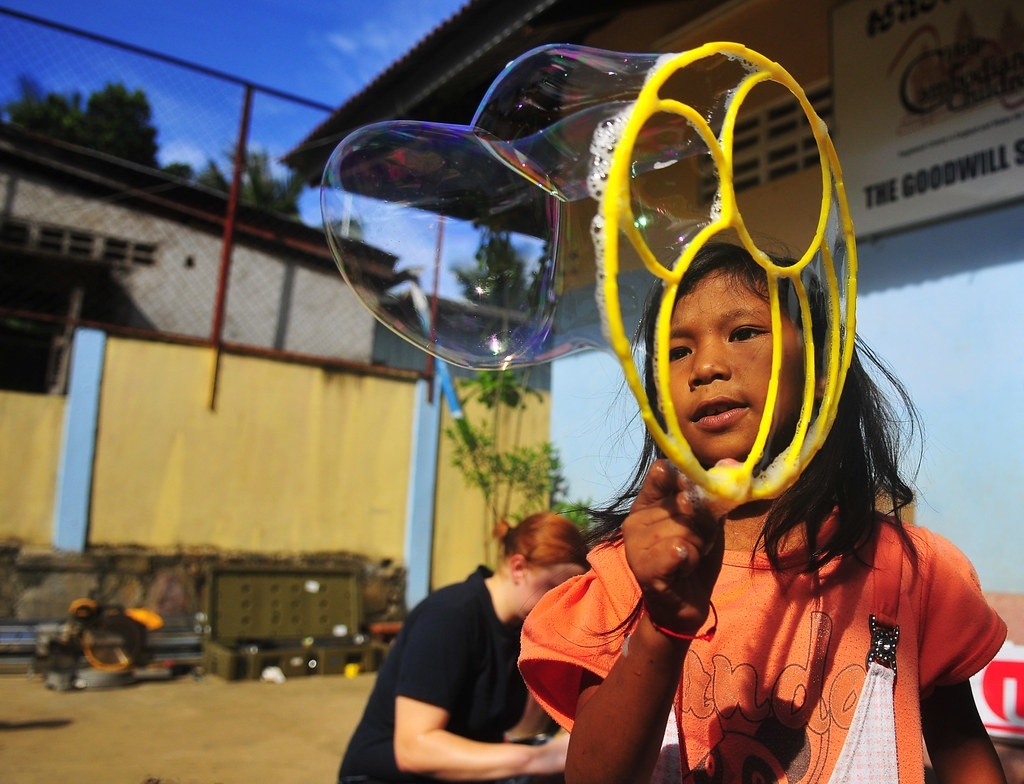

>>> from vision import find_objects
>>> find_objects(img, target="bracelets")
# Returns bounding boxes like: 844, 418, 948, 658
643, 600, 718, 642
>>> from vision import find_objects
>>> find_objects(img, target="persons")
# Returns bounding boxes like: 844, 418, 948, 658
339, 513, 592, 784
517, 242, 1007, 784
